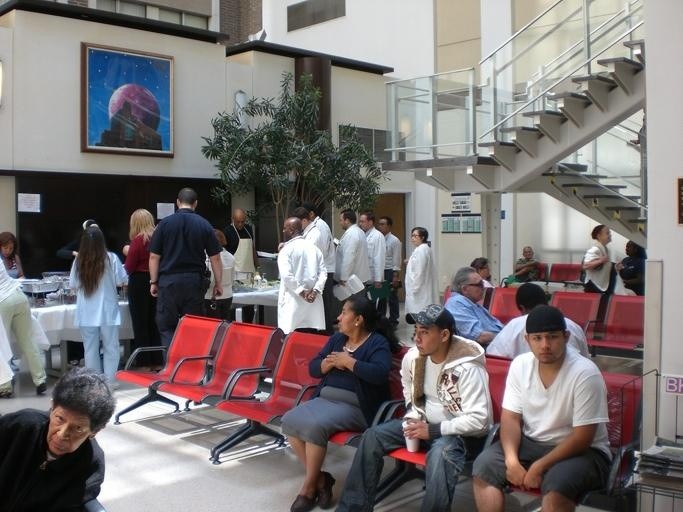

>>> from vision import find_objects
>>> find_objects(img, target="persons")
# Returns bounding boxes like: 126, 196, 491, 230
0, 232, 47, 396
445, 257, 503, 343
616, 241, 645, 297
0, 368, 117, 512
118, 189, 223, 373
278, 202, 335, 336
333, 208, 401, 326
487, 283, 592, 360
405, 227, 437, 339
515, 246, 540, 282
60, 219, 129, 389
281, 294, 393, 512
583, 226, 612, 314
205, 209, 261, 320
473, 305, 612, 511
336, 305, 495, 511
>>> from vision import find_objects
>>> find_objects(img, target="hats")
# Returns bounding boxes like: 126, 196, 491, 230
404, 303, 457, 333
523, 304, 568, 334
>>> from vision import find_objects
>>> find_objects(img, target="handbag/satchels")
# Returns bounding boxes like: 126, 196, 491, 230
579, 254, 618, 295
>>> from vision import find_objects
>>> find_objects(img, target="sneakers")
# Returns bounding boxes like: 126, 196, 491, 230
0, 390, 12, 399
35, 383, 47, 394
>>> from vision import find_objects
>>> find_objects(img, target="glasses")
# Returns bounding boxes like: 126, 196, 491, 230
465, 279, 484, 288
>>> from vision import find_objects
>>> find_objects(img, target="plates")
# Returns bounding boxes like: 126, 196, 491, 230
512, 281, 565, 288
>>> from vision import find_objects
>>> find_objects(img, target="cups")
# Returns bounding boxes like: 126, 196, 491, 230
403, 421, 423, 453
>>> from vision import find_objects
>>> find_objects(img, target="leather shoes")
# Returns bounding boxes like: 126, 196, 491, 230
289, 487, 317, 512
316, 470, 336, 508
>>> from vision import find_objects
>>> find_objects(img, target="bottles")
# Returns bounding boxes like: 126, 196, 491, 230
246, 267, 280, 290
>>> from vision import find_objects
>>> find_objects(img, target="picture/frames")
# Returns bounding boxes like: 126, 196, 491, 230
81, 42, 176, 158
